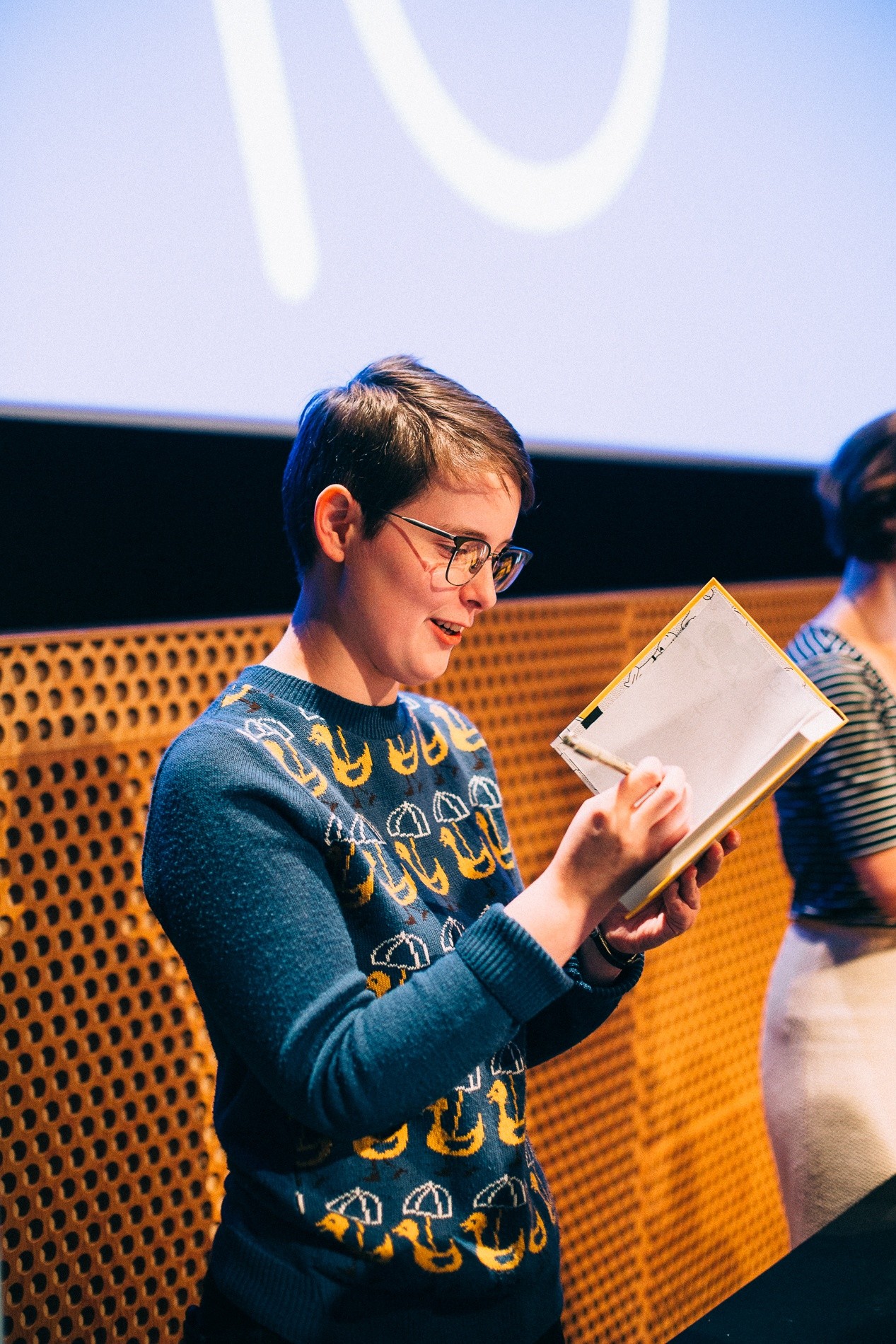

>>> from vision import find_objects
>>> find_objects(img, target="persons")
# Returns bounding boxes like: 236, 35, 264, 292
760, 408, 896, 1251
141, 352, 741, 1344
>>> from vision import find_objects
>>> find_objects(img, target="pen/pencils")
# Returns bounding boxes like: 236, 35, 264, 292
562, 732, 661, 788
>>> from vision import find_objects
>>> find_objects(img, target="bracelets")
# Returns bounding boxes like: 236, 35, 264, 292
588, 925, 637, 969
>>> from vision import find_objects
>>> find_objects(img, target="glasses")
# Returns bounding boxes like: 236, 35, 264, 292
355, 500, 533, 594
